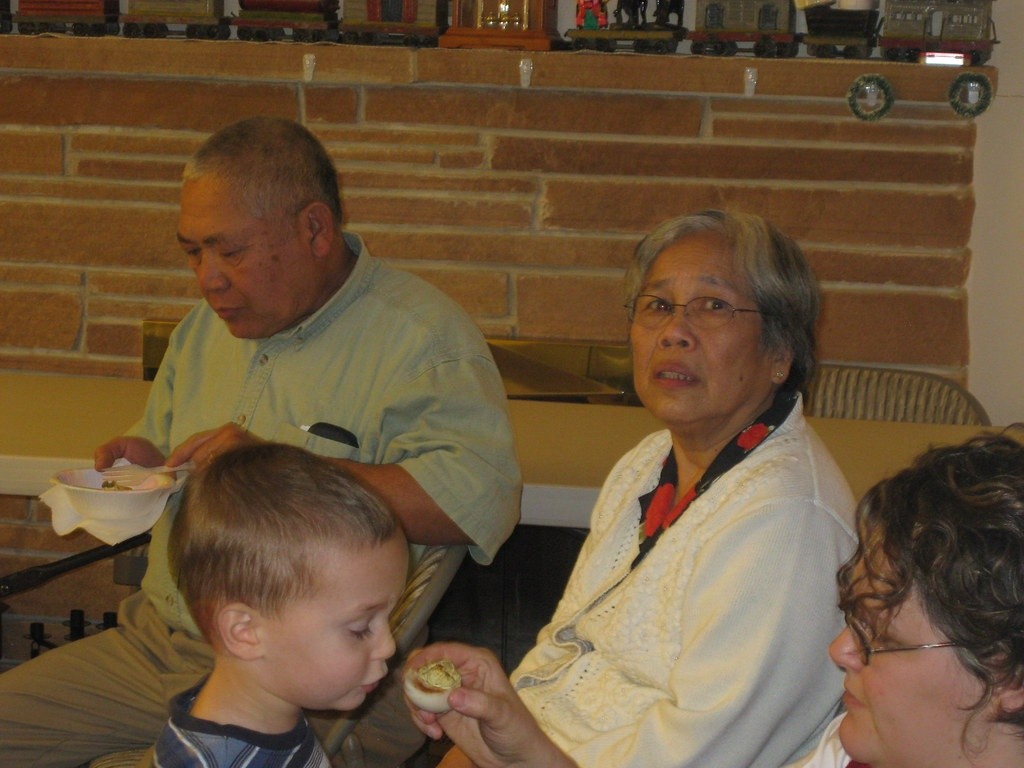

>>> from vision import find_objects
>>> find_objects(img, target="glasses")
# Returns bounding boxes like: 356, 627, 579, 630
623, 295, 764, 329
844, 614, 1000, 665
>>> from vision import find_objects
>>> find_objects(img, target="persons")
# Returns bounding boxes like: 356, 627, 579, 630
151, 445, 412, 768
401, 434, 1024, 768
430, 207, 857, 768
0, 115, 524, 768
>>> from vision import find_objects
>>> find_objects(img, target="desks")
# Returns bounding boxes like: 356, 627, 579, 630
0, 368, 1024, 767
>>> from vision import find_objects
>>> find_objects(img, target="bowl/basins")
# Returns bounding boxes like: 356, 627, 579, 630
50, 469, 161, 520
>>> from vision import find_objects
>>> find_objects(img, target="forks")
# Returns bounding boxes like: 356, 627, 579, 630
101, 465, 190, 486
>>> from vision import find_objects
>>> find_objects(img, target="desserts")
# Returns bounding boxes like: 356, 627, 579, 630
404, 659, 462, 714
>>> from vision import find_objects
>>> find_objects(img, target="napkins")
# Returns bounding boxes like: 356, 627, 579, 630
39, 458, 193, 546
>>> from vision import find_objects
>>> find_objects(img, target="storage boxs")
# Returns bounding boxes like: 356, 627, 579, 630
804, 5, 879, 39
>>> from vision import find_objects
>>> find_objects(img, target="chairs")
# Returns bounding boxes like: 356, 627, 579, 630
0, 492, 465, 767
805, 363, 995, 426
